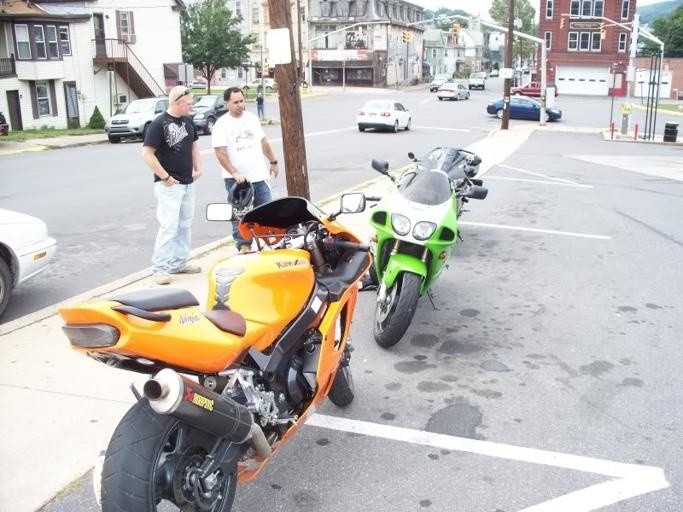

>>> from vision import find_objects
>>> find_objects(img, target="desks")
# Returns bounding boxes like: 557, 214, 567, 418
405, 14, 547, 126
561, 13, 664, 107
305, 20, 390, 95
561, 18, 567, 29
513, 17, 523, 86
599, 23, 605, 33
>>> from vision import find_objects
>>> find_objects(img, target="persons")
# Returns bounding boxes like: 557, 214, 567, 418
140, 84, 203, 286
255, 93, 264, 119
209, 86, 279, 254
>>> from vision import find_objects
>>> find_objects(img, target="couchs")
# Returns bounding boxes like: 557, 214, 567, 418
453, 24, 460, 38
407, 33, 410, 40
402, 32, 407, 43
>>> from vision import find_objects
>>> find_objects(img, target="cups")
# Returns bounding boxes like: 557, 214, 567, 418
175, 89, 191, 101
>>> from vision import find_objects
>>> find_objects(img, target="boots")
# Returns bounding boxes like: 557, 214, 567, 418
430, 74, 453, 90
469, 72, 486, 89
185, 93, 233, 136
103, 95, 173, 143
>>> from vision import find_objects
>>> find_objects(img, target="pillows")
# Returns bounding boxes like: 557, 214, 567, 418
228, 180, 255, 219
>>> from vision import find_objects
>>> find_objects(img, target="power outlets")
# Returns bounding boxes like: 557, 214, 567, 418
512, 82, 559, 97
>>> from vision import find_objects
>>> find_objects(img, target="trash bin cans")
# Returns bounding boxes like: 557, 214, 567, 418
162, 175, 170, 183
269, 160, 278, 165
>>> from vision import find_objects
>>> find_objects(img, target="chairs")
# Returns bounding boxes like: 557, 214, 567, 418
178, 265, 201, 273
154, 274, 168, 284
240, 245, 249, 254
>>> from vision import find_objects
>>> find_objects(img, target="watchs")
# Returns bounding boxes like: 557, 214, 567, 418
55, 191, 373, 511
367, 145, 491, 348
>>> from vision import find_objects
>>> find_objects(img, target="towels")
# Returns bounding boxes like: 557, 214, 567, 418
663, 123, 679, 142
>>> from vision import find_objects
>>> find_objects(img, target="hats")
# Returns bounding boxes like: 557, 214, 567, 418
438, 82, 470, 101
241, 78, 278, 89
489, 69, 498, 77
357, 98, 415, 132
487, 94, 562, 124
0, 208, 58, 317
